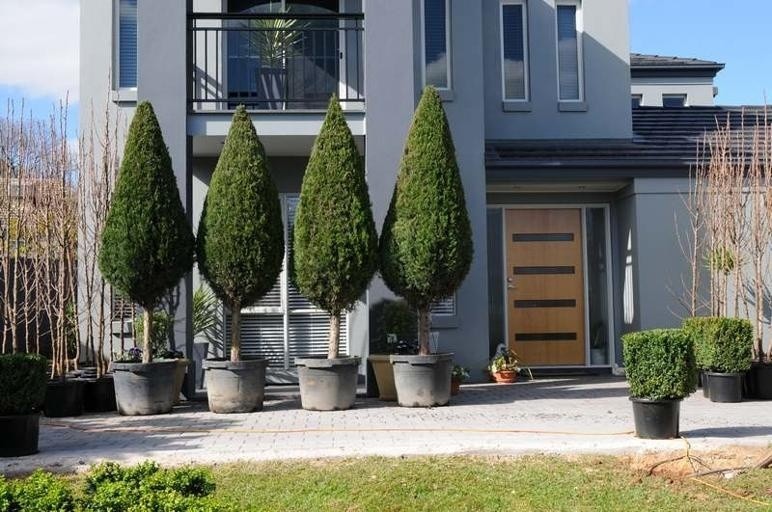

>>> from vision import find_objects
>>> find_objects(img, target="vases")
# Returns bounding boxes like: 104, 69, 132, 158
492, 371, 518, 384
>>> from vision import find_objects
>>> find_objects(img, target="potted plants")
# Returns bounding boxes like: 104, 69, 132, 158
620, 313, 756, 440
236, 2, 313, 110
193, 103, 287, 414
451, 365, 469, 398
287, 92, 379, 411
367, 297, 418, 401
377, 83, 474, 410
1, 349, 49, 458
99, 99, 195, 414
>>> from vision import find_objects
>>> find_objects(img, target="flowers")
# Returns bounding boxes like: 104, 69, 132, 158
486, 355, 521, 374
